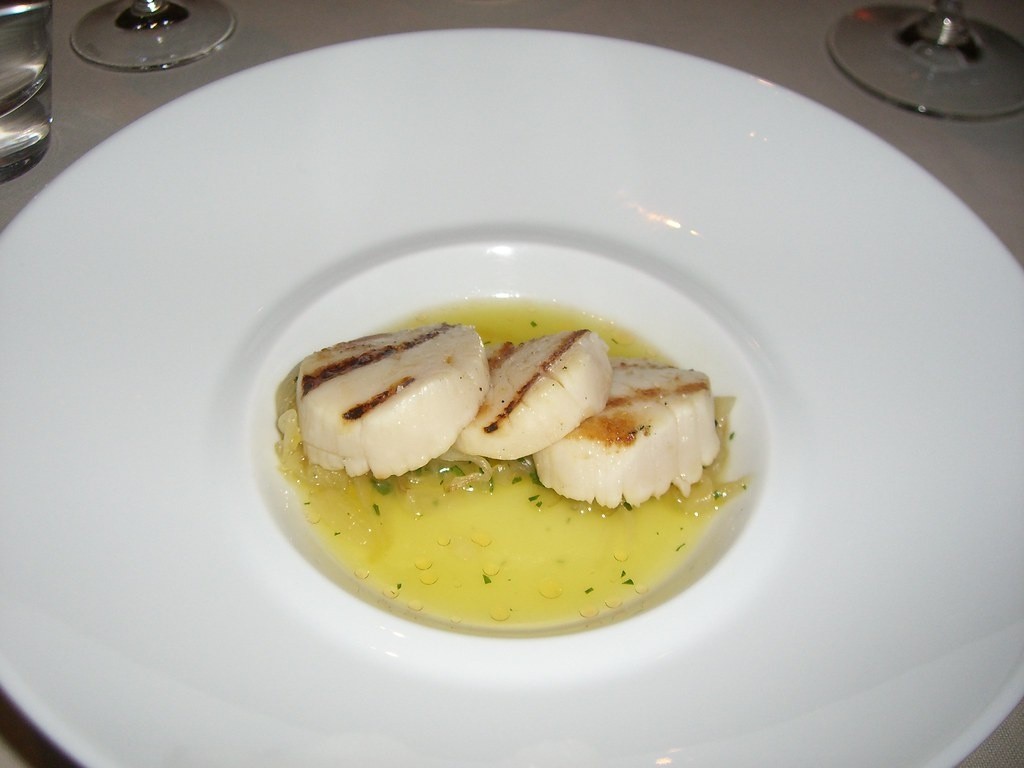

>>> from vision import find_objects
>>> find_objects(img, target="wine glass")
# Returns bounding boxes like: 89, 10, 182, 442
828, 0, 1024, 118
70, 0, 236, 70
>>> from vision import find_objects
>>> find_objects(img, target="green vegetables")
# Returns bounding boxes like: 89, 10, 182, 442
306, 457, 722, 595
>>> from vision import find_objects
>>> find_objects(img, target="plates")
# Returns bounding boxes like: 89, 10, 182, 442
0, 30, 1022, 768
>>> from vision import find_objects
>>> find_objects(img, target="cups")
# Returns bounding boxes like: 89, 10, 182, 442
0, 0, 51, 183
